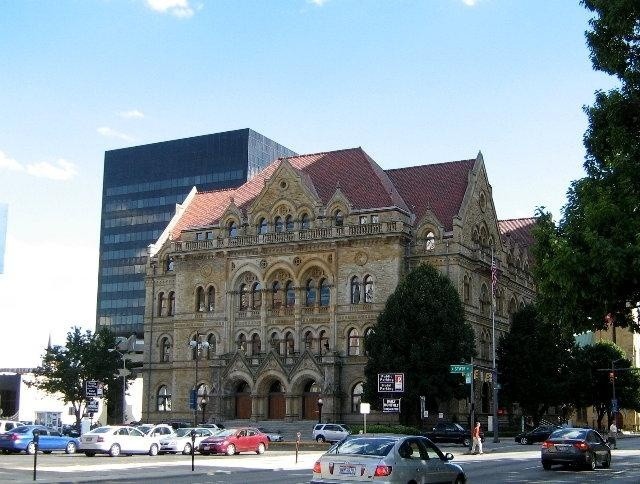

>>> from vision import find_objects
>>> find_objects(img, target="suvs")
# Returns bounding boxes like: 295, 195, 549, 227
312, 422, 354, 442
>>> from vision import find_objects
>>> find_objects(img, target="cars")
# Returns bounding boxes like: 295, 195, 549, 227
514, 424, 565, 444
310, 434, 468, 484
540, 427, 612, 470
198, 426, 269, 456
158, 428, 214, 455
0, 418, 285, 443
422, 420, 485, 445
0, 424, 80, 454
78, 425, 161, 456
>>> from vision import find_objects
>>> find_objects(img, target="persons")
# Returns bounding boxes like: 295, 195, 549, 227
470, 420, 484, 454
607, 419, 619, 449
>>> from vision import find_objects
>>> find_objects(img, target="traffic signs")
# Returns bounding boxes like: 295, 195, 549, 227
448, 364, 473, 372
383, 398, 401, 413
378, 373, 404, 392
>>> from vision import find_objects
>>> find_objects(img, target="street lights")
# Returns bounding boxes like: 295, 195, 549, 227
318, 398, 323, 423
491, 304, 500, 444
108, 348, 126, 425
189, 330, 210, 470
610, 355, 636, 424
201, 398, 207, 424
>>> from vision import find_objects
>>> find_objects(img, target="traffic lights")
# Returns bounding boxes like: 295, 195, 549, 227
474, 369, 480, 379
485, 372, 492, 382
610, 372, 615, 382
497, 409, 504, 416
